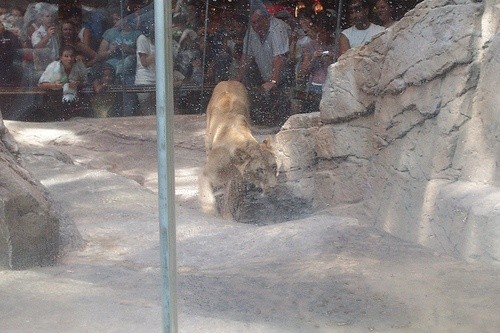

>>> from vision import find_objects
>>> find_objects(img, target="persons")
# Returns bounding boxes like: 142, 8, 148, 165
1, 1, 407, 127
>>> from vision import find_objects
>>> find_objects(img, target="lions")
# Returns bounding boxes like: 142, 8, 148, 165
198, 79, 279, 218
